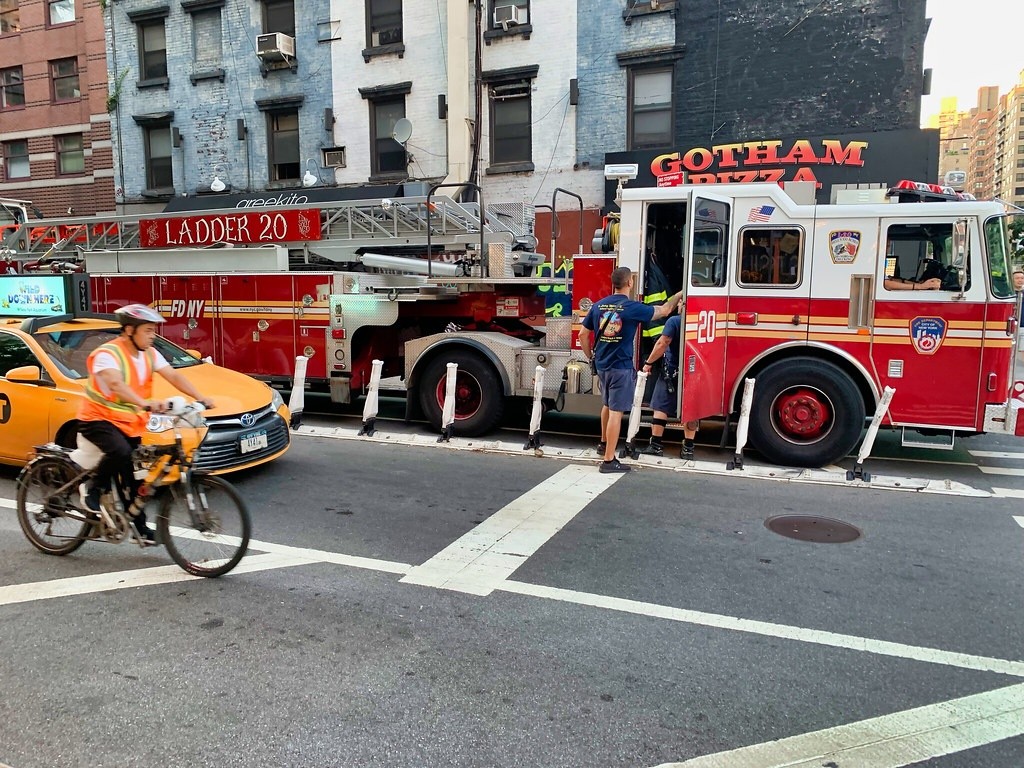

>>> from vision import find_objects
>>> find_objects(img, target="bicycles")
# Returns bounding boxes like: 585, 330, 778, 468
16, 402, 252, 577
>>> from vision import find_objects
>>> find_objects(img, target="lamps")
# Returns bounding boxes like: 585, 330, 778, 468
303, 158, 338, 187
210, 163, 234, 194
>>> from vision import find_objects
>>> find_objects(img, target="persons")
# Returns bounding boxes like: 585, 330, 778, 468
642, 295, 696, 461
1013, 271, 1024, 361
578, 267, 683, 473
884, 239, 941, 290
76, 303, 215, 546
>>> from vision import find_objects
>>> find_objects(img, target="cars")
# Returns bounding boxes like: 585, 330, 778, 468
0, 312, 292, 492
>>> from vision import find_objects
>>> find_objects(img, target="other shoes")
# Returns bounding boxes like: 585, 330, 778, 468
599, 455, 631, 473
78, 479, 102, 514
680, 440, 695, 459
128, 524, 157, 545
597, 441, 618, 455
641, 437, 663, 456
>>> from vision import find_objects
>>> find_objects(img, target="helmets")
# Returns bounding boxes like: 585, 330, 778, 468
114, 304, 167, 326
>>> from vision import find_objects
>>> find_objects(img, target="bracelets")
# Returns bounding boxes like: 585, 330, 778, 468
644, 360, 654, 365
912, 283, 915, 290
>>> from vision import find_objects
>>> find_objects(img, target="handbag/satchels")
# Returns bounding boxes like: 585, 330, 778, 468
665, 366, 679, 398
589, 356, 597, 375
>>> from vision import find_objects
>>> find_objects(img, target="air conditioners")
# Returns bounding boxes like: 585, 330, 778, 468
255, 31, 295, 71
495, 5, 519, 30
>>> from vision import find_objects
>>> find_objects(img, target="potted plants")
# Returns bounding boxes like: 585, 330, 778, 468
106, 68, 129, 113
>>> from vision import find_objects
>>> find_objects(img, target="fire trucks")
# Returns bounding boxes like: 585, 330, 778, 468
0, 179, 1021, 470
